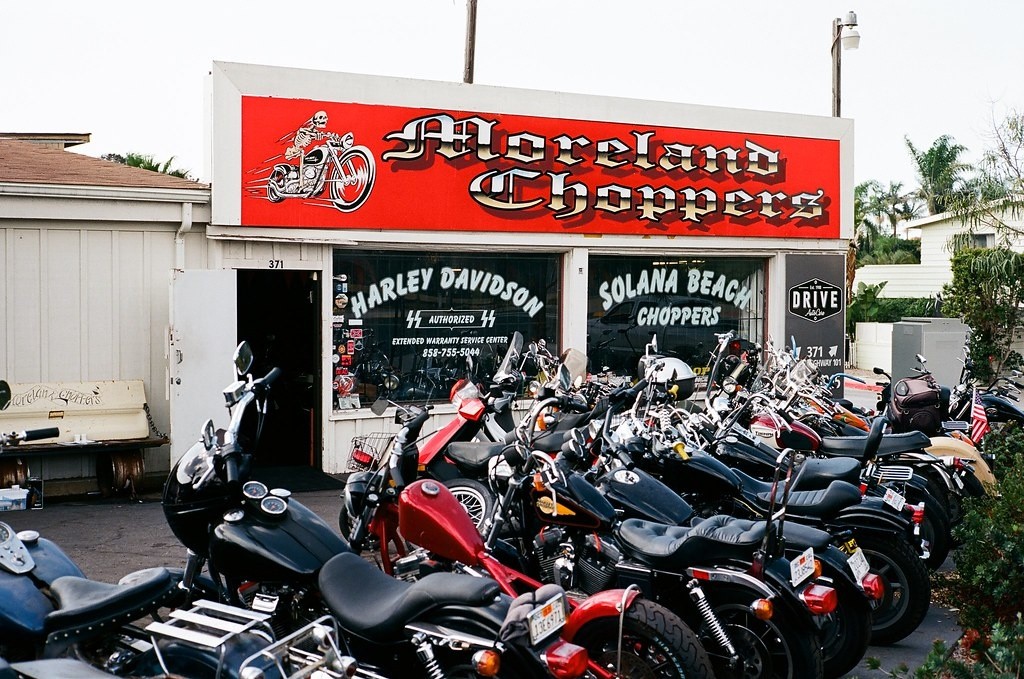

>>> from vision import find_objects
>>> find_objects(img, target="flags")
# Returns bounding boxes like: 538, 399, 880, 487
970, 387, 988, 443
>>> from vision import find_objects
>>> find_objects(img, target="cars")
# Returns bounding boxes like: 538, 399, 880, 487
458, 308, 558, 354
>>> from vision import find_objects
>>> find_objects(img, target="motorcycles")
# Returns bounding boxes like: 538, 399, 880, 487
343, 324, 556, 402
589, 316, 705, 370
0, 330, 1023, 679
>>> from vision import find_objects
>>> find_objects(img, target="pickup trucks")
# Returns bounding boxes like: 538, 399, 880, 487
587, 296, 742, 361
